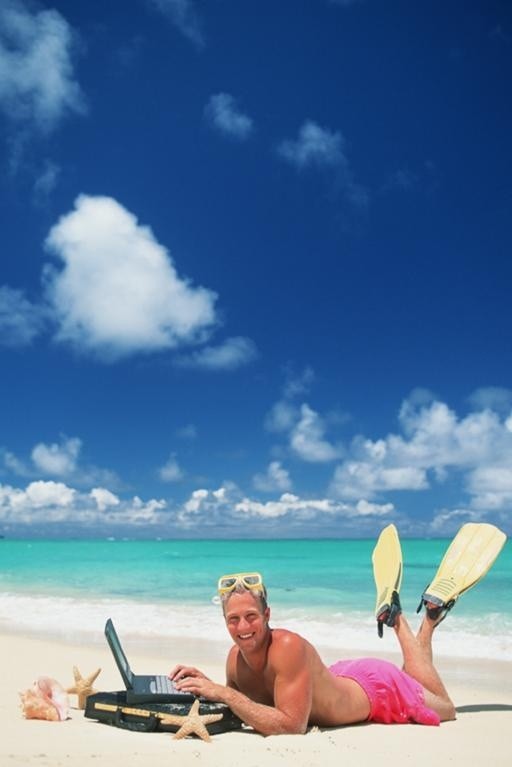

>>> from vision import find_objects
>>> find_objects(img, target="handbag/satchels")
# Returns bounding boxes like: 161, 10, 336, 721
84, 690, 242, 737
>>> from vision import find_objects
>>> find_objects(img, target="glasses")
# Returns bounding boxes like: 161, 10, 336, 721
217, 573, 263, 593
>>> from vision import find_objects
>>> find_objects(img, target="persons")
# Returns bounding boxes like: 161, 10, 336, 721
167, 521, 509, 738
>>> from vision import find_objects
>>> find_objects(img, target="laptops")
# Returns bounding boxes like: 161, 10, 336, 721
104, 617, 197, 704
